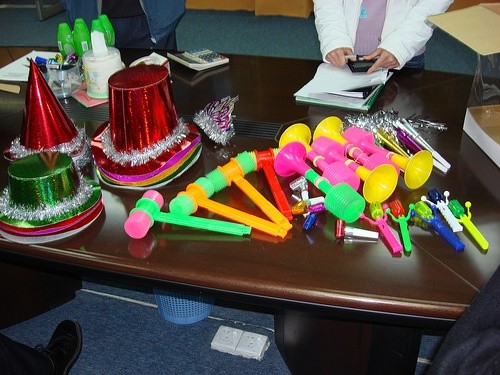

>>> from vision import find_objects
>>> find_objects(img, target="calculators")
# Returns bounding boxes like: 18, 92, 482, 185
166, 47, 230, 72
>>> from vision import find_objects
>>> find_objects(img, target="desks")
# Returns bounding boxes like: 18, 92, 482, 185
0, 43, 500, 320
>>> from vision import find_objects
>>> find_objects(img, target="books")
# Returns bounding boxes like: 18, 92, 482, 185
463, 105, 500, 168
294, 71, 394, 111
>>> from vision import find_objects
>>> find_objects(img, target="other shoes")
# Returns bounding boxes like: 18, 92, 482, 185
35, 320, 83, 375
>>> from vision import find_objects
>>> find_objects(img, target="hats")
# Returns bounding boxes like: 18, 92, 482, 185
0, 58, 90, 170
0, 151, 104, 244
91, 64, 202, 191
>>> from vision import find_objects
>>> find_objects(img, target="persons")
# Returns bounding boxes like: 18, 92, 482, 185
0, 320, 81, 375
62, 0, 185, 50
313, 0, 454, 73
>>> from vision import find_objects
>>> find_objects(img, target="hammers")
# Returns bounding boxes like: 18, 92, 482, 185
247, 147, 294, 221
204, 150, 294, 232
168, 176, 288, 239
123, 189, 252, 239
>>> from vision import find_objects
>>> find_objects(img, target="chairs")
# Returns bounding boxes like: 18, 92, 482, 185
274, 308, 421, 375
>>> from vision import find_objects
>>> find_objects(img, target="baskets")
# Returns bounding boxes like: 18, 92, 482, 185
153, 293, 211, 324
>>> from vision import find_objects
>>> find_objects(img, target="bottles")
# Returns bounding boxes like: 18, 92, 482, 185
57, 13, 115, 58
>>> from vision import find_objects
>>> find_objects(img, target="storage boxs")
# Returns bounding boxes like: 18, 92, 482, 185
463, 105, 500, 166
428, 4, 500, 57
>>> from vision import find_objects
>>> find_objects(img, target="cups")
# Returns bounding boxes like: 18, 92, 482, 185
44, 60, 80, 99
